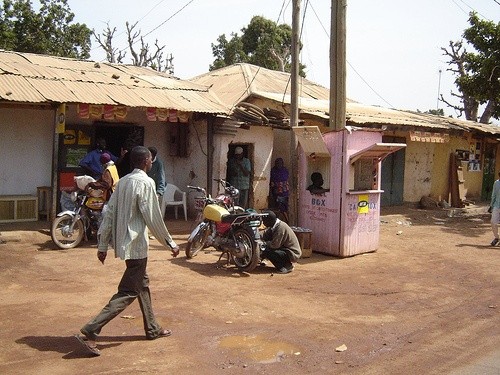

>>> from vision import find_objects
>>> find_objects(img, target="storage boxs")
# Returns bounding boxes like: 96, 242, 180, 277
0, 194, 38, 223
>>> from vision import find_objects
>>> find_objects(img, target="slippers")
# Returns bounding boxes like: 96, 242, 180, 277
278, 266, 294, 274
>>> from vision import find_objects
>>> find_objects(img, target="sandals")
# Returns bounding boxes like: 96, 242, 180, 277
159, 326, 173, 336
74, 331, 101, 356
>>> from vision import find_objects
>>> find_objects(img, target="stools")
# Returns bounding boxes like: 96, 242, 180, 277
36, 186, 52, 221
293, 229, 312, 257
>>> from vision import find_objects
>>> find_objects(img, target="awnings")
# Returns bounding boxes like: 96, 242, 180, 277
350, 143, 408, 165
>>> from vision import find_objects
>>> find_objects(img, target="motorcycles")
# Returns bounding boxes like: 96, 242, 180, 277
184, 185, 270, 273
49, 181, 112, 250
188, 178, 247, 250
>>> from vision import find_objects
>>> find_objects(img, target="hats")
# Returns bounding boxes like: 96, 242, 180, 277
100, 153, 111, 163
234, 147, 243, 154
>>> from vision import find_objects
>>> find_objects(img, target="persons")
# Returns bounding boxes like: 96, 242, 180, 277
488, 172, 500, 248
260, 210, 303, 274
226, 146, 251, 209
307, 172, 324, 190
74, 146, 180, 356
77, 138, 168, 238
271, 157, 290, 219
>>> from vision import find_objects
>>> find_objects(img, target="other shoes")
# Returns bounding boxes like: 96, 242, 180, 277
491, 238, 500, 246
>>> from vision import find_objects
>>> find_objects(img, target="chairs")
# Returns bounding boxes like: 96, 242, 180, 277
163, 184, 188, 221
